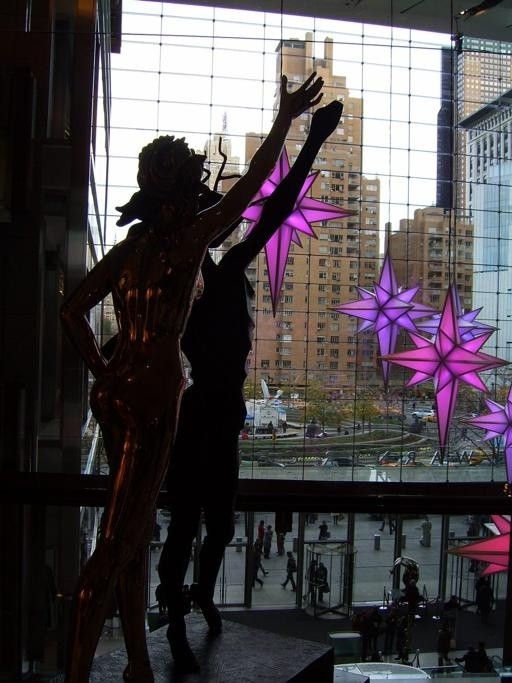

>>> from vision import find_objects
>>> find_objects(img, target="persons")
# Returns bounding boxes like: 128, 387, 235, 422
59, 72, 325, 683
101, 100, 343, 674
242, 419, 289, 445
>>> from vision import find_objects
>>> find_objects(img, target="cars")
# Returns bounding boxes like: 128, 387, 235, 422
321, 458, 364, 467
412, 409, 436, 418
